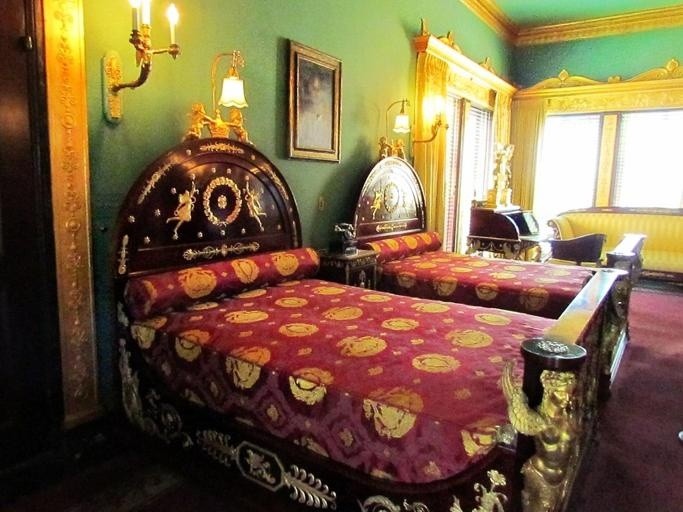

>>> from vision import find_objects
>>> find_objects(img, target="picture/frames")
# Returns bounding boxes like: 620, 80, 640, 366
286, 38, 342, 163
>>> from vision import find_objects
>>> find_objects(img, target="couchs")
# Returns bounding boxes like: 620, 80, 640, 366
547, 206, 683, 286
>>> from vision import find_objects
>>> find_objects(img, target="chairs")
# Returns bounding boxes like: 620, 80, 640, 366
549, 233, 605, 267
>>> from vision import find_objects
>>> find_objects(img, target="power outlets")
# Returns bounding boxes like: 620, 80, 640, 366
319, 196, 325, 211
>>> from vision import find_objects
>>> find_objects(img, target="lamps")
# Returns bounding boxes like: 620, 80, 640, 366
104, 0, 182, 124
387, 100, 409, 134
415, 99, 448, 144
211, 50, 249, 110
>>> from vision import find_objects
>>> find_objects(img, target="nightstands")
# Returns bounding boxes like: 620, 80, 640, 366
316, 249, 378, 290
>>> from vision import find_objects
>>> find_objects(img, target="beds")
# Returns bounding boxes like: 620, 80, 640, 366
114, 102, 630, 512
352, 137, 647, 398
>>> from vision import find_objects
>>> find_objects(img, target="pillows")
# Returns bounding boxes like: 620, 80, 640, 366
364, 232, 443, 263
124, 247, 320, 321
